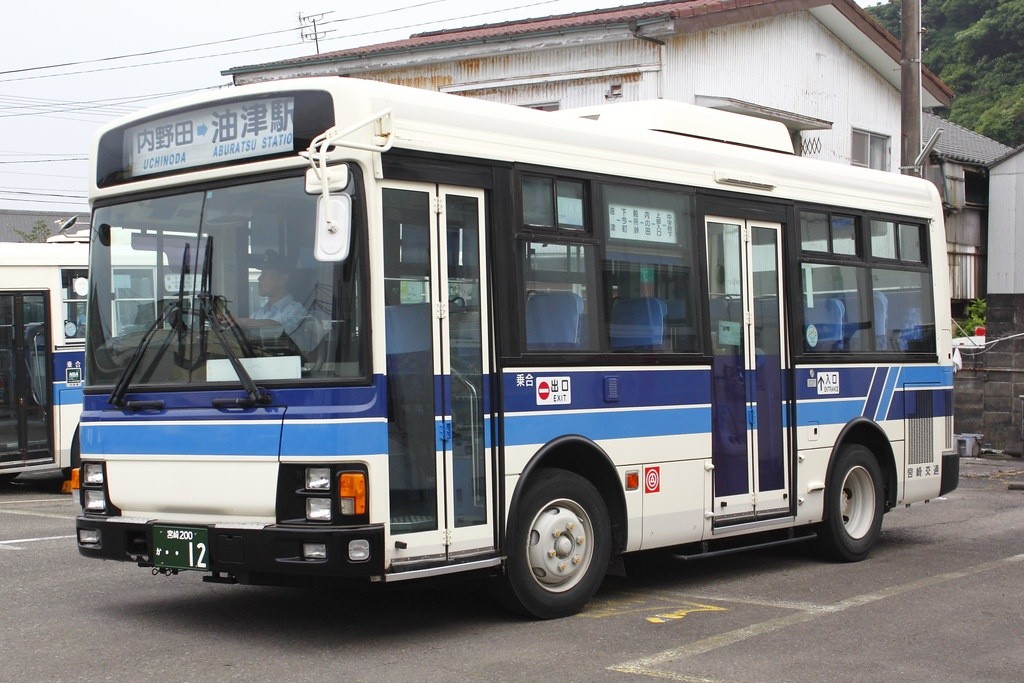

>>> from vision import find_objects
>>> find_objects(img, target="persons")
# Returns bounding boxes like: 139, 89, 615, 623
214, 249, 325, 361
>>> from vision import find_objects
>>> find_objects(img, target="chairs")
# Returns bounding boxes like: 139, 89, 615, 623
387, 284, 922, 371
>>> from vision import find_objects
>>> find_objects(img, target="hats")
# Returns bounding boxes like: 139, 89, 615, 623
255, 248, 293, 274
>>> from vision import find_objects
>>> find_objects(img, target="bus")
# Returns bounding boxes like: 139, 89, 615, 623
0, 226, 265, 485
77, 76, 960, 623
